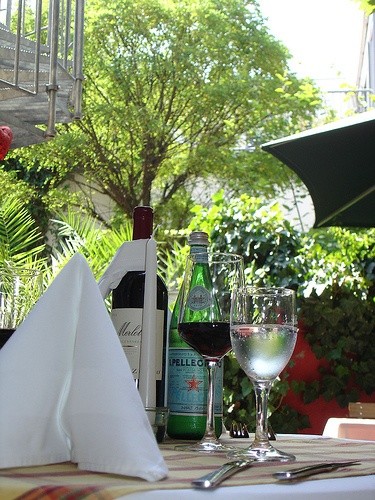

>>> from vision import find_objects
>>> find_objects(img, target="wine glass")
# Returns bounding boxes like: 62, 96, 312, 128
174, 251, 247, 459
225, 286, 297, 464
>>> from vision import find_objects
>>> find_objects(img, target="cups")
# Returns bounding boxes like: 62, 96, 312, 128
146, 407, 168, 444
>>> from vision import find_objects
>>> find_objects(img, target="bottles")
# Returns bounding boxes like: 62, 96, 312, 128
112, 206, 169, 443
165, 232, 223, 440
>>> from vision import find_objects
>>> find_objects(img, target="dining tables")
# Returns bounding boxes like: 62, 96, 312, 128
0, 433, 375, 500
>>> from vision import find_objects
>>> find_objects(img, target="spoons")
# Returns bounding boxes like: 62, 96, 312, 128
192, 460, 252, 488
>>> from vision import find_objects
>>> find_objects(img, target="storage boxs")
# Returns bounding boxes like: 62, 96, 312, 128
348, 401, 375, 418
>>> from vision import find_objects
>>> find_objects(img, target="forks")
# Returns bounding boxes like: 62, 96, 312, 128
230, 423, 249, 438
267, 425, 277, 440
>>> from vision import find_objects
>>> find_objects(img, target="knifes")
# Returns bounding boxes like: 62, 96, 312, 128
273, 460, 358, 480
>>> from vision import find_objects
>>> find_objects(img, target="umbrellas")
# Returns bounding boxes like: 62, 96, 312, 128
260, 111, 374, 231
